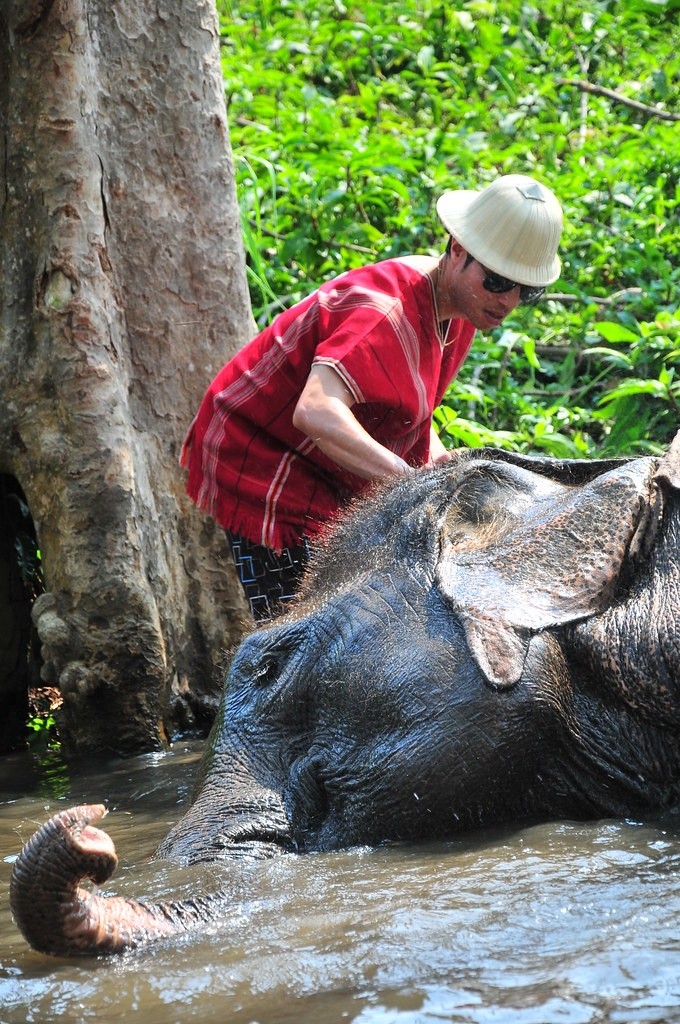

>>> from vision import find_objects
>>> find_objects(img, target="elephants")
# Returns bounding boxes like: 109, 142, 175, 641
7, 426, 680, 958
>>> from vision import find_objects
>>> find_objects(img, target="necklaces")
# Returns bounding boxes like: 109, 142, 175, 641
422, 268, 441, 342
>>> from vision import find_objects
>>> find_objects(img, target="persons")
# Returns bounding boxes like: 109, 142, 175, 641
178, 175, 562, 626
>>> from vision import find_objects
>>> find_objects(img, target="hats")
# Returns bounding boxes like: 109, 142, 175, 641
436, 174, 563, 287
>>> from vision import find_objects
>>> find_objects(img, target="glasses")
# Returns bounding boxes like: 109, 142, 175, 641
479, 262, 546, 304
